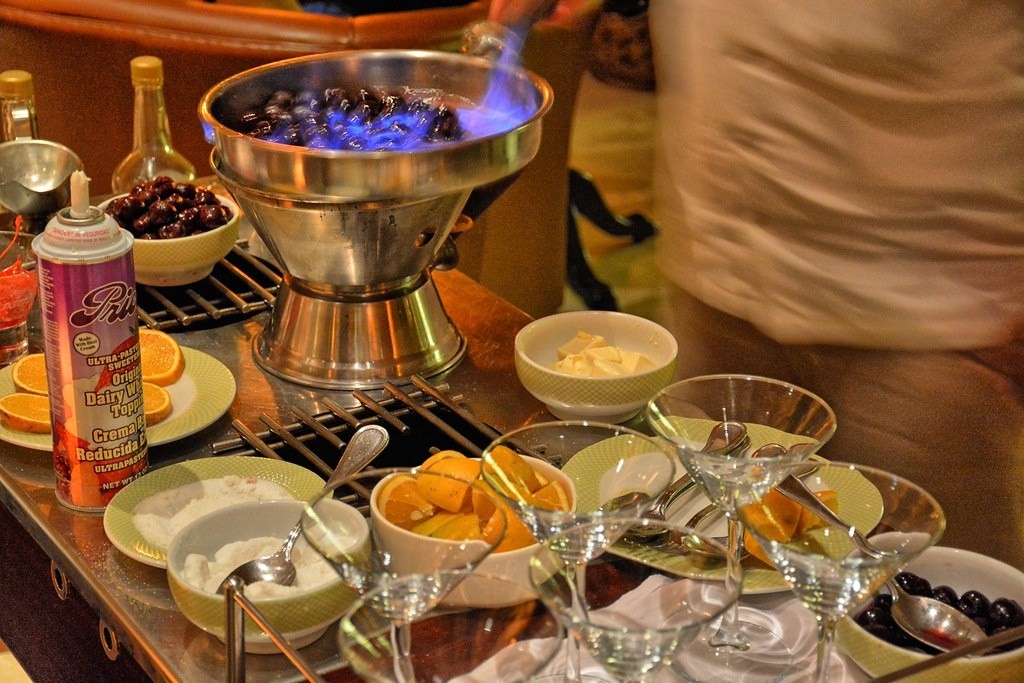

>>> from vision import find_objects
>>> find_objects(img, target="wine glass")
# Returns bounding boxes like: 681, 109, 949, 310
300, 467, 507, 683
650, 373, 837, 683
736, 462, 945, 683
482, 420, 676, 683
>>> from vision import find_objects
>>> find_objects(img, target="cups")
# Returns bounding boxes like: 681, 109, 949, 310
342, 573, 565, 683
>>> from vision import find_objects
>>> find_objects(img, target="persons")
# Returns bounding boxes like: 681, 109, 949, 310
488, 0, 1024, 571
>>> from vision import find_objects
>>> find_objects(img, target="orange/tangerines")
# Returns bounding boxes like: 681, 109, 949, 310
377, 444, 571, 554
733, 489, 841, 570
0, 328, 186, 434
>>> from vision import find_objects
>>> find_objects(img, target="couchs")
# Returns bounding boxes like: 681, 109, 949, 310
0, 0, 603, 321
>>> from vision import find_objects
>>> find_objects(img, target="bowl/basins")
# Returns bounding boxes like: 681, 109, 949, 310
166, 500, 373, 654
99, 193, 243, 286
513, 310, 678, 424
371, 454, 576, 608
837, 533, 1024, 683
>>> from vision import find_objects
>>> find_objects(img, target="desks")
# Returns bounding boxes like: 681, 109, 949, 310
0, 172, 650, 683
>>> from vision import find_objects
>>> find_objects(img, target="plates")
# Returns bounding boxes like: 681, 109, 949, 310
103, 455, 334, 570
0, 345, 237, 448
202, 48, 555, 197
561, 416, 884, 594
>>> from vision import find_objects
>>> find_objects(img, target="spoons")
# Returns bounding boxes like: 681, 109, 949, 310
217, 425, 390, 594
601, 420, 993, 656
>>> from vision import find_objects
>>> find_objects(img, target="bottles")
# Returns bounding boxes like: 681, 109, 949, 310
0, 70, 40, 140
112, 56, 195, 194
31, 171, 148, 512
